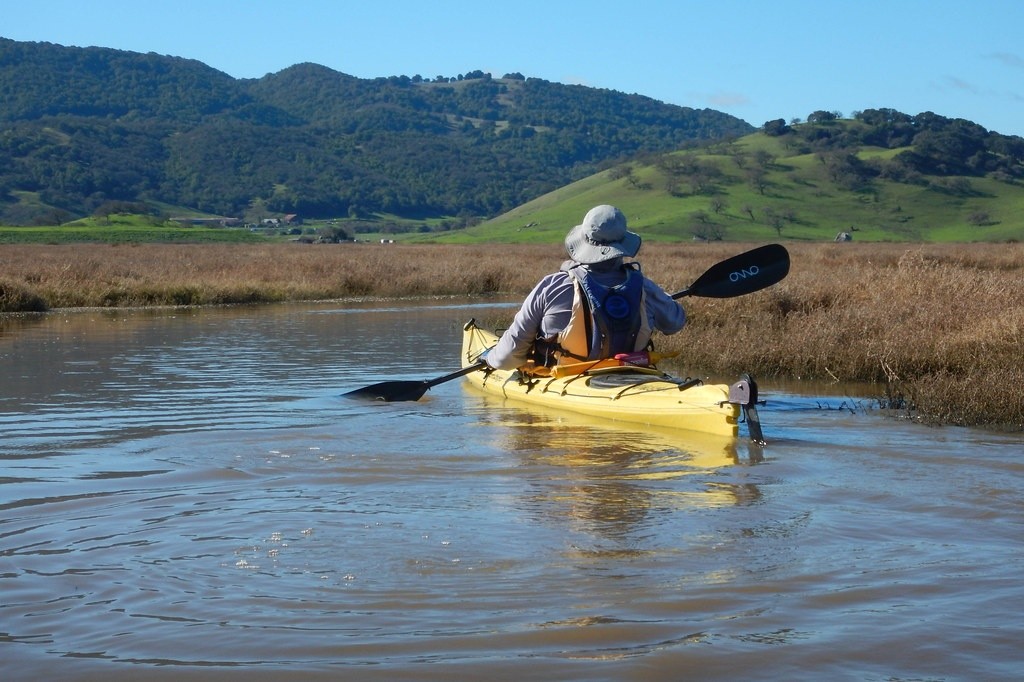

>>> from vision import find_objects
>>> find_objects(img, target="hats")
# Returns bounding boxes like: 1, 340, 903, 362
564, 203, 642, 264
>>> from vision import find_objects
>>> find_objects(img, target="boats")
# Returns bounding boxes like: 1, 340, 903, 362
461, 317, 767, 440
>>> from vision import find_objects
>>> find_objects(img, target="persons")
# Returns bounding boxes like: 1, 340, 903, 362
486, 204, 685, 371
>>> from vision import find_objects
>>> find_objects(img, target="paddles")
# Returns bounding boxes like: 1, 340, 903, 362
340, 244, 789, 403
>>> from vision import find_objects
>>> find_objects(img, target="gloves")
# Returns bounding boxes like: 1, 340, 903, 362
476, 351, 497, 373
